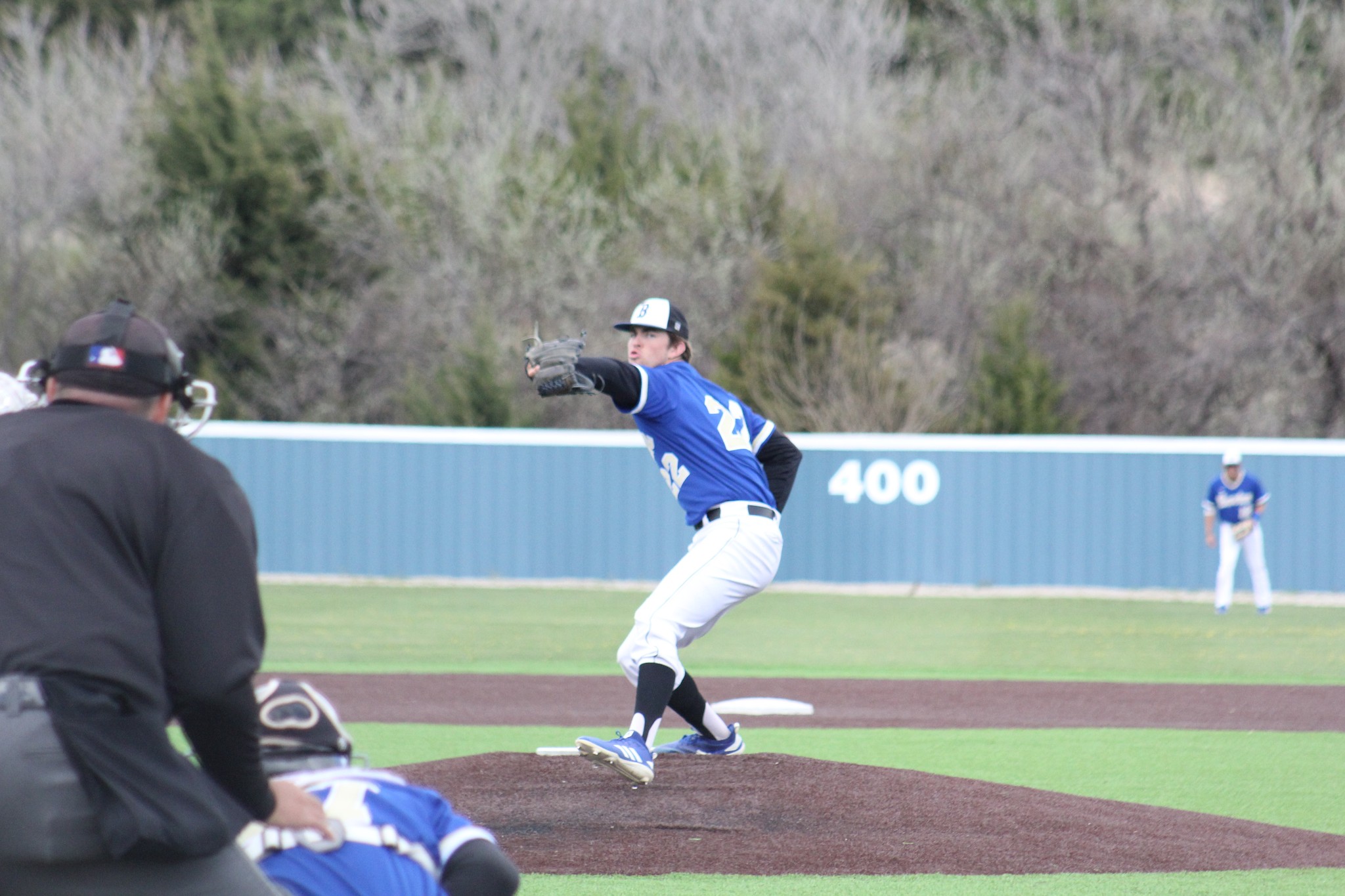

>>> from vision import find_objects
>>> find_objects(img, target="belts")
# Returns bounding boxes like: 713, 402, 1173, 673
695, 505, 775, 533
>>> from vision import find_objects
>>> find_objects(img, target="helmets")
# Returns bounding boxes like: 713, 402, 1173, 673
252, 676, 352, 755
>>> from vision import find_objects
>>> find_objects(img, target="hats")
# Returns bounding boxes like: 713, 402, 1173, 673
1222, 450, 1242, 466
55, 309, 182, 372
614, 298, 688, 340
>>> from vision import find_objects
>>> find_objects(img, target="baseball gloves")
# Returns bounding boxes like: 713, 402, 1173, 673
522, 331, 606, 398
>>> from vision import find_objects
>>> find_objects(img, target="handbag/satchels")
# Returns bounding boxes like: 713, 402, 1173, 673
39, 675, 252, 861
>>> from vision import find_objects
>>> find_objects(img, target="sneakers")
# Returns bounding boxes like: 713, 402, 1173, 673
651, 722, 745, 756
576, 730, 657, 784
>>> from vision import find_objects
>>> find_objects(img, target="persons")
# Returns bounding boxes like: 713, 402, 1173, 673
525, 297, 802, 785
251, 678, 519, 896
1201, 454, 1273, 613
0, 297, 335, 896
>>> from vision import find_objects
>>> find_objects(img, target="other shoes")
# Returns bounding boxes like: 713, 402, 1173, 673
1259, 608, 1269, 614
1215, 605, 1227, 616
